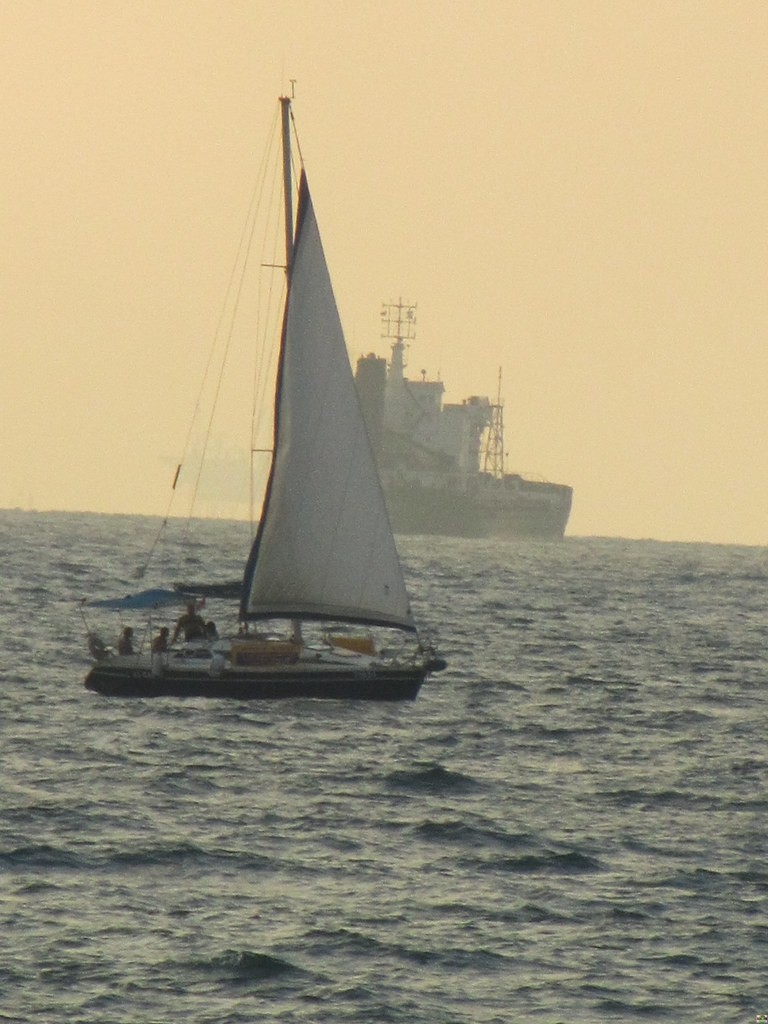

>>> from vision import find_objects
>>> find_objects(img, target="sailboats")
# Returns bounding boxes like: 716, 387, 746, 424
78, 79, 446, 702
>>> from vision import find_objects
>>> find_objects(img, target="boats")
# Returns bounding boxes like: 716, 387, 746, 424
355, 298, 573, 542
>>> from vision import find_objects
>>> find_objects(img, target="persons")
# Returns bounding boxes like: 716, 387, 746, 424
151, 627, 169, 651
173, 603, 204, 642
119, 627, 135, 654
207, 622, 219, 641
